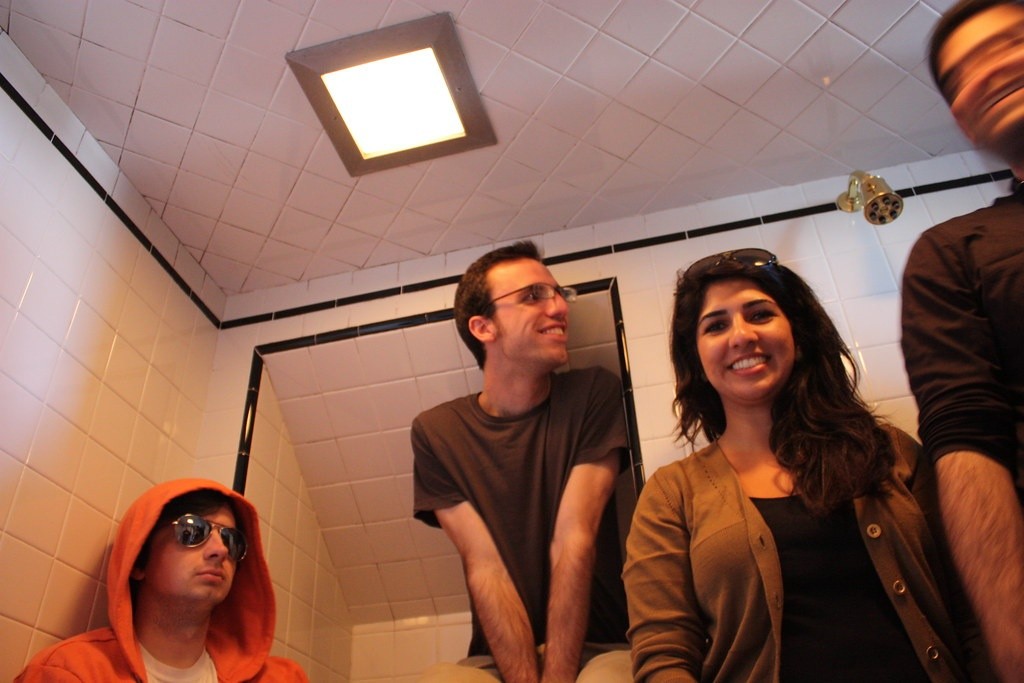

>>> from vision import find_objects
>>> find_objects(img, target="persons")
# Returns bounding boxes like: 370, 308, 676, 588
15, 478, 309, 683
621, 247, 995, 683
410, 242, 638, 682
901, 0, 1024, 683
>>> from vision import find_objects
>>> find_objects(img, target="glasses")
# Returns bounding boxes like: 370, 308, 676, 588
154, 515, 247, 562
481, 283, 577, 314
684, 248, 779, 286
